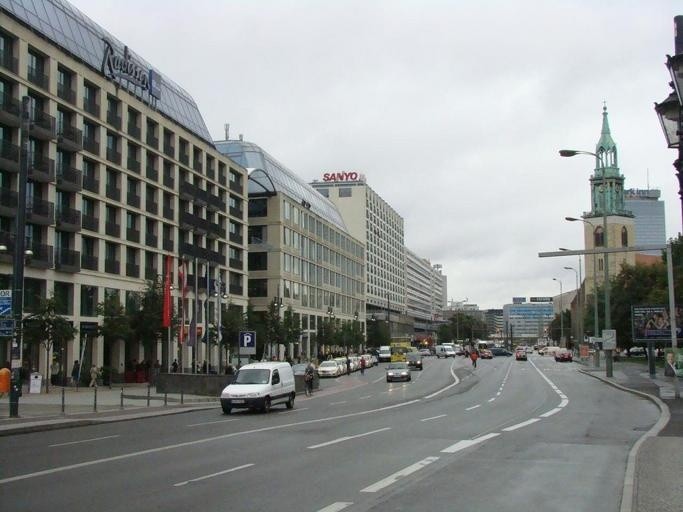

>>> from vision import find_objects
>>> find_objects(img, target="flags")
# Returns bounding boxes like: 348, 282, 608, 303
163, 254, 223, 346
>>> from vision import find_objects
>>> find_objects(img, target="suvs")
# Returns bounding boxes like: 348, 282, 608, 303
405, 352, 425, 370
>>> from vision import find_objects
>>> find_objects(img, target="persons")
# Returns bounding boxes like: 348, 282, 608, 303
71, 360, 80, 392
272, 348, 378, 396
126, 358, 201, 375
641, 306, 683, 330
89, 365, 98, 388
50, 359, 60, 387
470, 351, 478, 368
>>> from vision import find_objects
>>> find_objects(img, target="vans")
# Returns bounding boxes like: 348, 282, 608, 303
539, 346, 560, 355
376, 345, 390, 362
220, 361, 297, 416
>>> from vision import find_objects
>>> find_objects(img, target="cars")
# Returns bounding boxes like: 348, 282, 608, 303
386, 362, 411, 382
316, 353, 378, 378
552, 350, 572, 362
419, 342, 539, 361
411, 347, 418, 352
589, 344, 667, 359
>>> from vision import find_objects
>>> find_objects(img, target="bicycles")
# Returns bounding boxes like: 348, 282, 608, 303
304, 372, 315, 397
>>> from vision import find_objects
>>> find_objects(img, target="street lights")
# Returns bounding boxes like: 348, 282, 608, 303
560, 246, 588, 360
152, 273, 174, 372
214, 272, 228, 375
326, 304, 335, 321
563, 216, 601, 367
650, 12, 682, 227
558, 148, 614, 374
564, 266, 581, 358
272, 282, 284, 360
384, 316, 389, 325
7, 94, 34, 419
370, 313, 376, 324
552, 276, 563, 349
354, 308, 360, 320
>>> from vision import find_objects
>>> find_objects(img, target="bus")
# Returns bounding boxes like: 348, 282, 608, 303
390, 336, 411, 363
